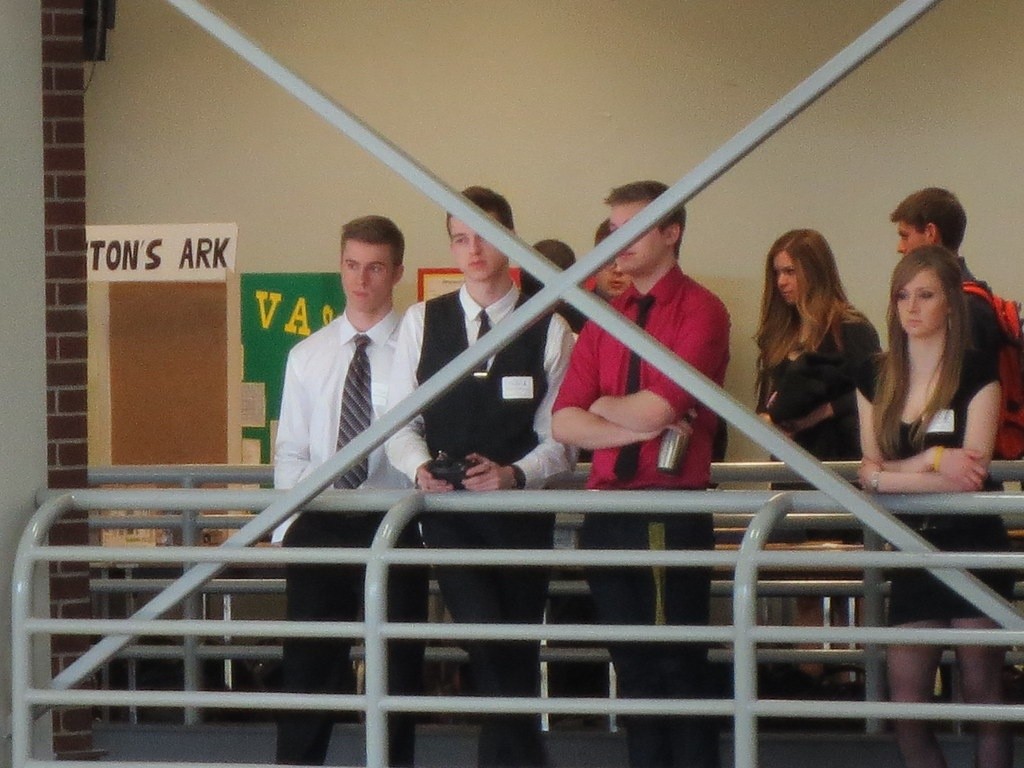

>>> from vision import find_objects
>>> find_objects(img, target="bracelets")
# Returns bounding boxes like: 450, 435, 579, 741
510, 464, 526, 489
871, 468, 882, 493
934, 446, 943, 471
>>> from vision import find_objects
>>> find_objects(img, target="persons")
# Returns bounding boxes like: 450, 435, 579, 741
520, 239, 578, 298
890, 187, 1003, 490
381, 187, 576, 768
568, 218, 632, 335
550, 180, 732, 768
272, 215, 429, 768
755, 228, 887, 733
857, 242, 1007, 768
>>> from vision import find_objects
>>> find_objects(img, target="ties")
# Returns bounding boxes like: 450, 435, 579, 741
613, 296, 654, 485
335, 334, 373, 489
472, 309, 489, 386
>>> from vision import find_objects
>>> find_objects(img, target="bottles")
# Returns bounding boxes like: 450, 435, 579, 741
657, 402, 700, 473
820, 663, 844, 701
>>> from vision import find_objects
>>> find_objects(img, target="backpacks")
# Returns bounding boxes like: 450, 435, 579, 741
957, 281, 1024, 461
